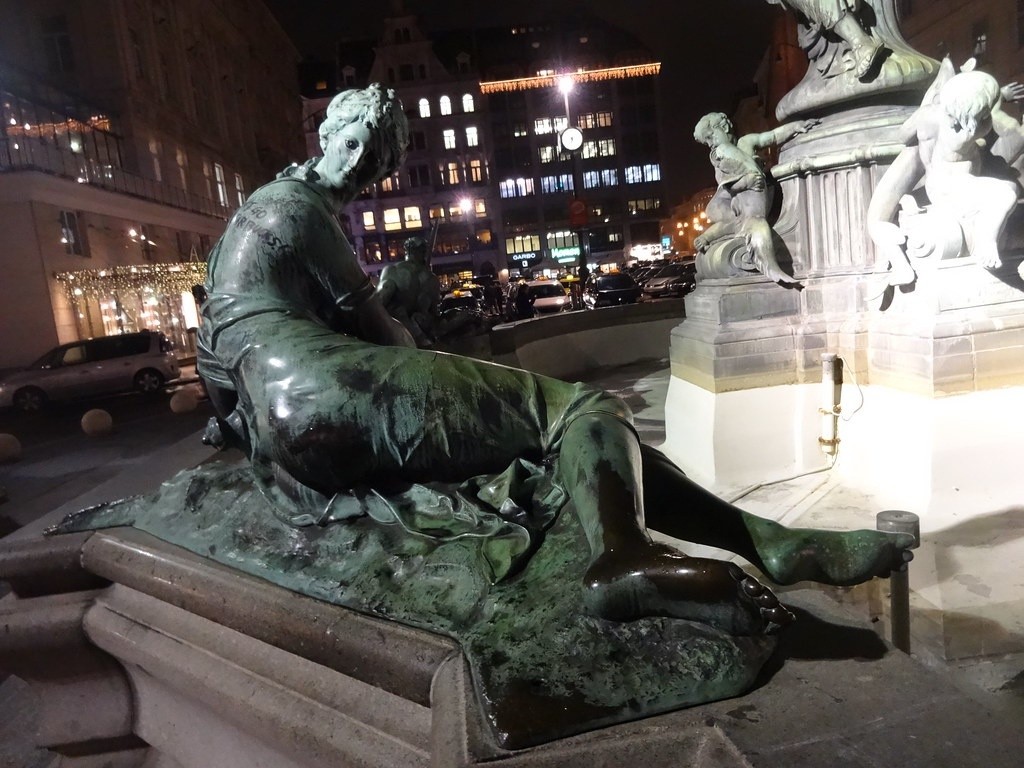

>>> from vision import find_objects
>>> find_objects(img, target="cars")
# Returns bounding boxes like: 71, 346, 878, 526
0, 329, 182, 414
439, 255, 696, 317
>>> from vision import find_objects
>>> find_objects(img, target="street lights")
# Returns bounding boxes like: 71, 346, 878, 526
456, 196, 478, 273
552, 68, 589, 269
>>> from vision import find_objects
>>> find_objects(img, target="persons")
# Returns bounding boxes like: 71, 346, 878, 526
767, 0, 884, 78
494, 281, 503, 314
515, 283, 537, 319
196, 81, 916, 636
693, 113, 819, 251
376, 236, 440, 343
897, 71, 1024, 281
